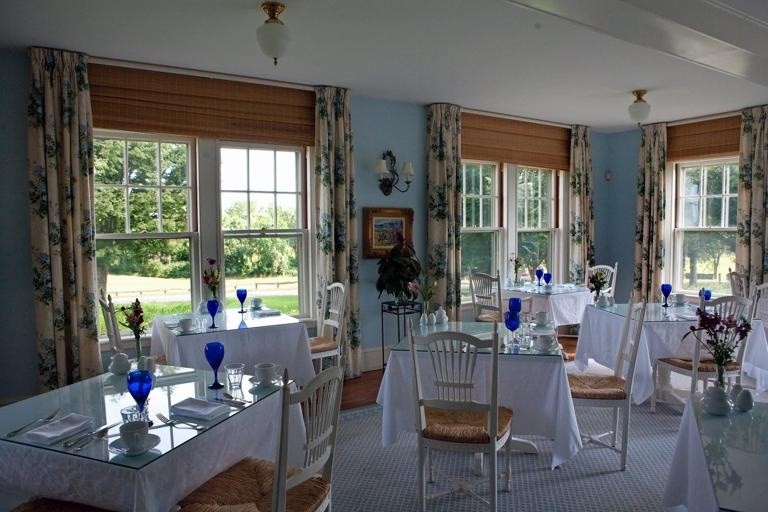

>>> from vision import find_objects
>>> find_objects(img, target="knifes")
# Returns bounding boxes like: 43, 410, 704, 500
73, 428, 108, 451
60, 420, 125, 448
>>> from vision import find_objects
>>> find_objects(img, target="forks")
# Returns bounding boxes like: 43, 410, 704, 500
4, 406, 62, 437
155, 411, 208, 432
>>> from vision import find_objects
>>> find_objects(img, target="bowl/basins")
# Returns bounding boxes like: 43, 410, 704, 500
178, 318, 193, 329
253, 363, 275, 387
119, 420, 149, 453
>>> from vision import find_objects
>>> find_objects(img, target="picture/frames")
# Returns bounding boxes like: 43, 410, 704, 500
363, 208, 414, 257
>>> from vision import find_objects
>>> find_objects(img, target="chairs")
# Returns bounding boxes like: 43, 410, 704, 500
583, 259, 619, 304
567, 292, 647, 471
467, 265, 510, 324
7, 497, 118, 512
169, 342, 349, 512
649, 287, 758, 414
728, 265, 748, 298
98, 287, 167, 365
408, 324, 514, 512
307, 281, 346, 387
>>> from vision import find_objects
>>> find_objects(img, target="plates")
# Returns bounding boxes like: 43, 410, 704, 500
250, 306, 264, 310
248, 375, 281, 387
529, 324, 555, 331
248, 385, 280, 395
529, 344, 563, 354
107, 433, 161, 457
110, 448, 162, 469
173, 327, 198, 333
671, 299, 690, 306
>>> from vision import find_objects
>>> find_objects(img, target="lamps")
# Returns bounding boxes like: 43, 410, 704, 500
254, 3, 289, 65
374, 151, 412, 196
628, 90, 651, 124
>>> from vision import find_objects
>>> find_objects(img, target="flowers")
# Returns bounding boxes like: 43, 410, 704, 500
682, 308, 754, 392
509, 252, 521, 287
407, 277, 439, 324
588, 271, 608, 302
118, 297, 146, 363
202, 257, 223, 299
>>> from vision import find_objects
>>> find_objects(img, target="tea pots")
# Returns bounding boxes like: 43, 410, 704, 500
698, 378, 734, 415
505, 276, 515, 288
597, 292, 611, 308
198, 299, 210, 314
435, 306, 448, 325
108, 348, 132, 377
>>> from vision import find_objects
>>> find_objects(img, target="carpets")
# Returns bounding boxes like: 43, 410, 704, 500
331, 361, 768, 512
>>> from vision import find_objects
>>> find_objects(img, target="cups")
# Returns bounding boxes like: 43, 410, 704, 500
508, 297, 522, 343
704, 289, 712, 302
675, 293, 685, 302
206, 299, 220, 329
203, 341, 224, 389
660, 283, 673, 307
251, 298, 262, 306
127, 368, 155, 427
531, 332, 555, 349
536, 311, 549, 325
239, 312, 248, 329
236, 288, 248, 314
536, 269, 543, 286
229, 388, 244, 400
504, 313, 521, 355
543, 272, 552, 289
224, 362, 245, 388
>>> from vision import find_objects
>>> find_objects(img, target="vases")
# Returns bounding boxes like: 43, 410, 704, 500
136, 338, 142, 362
423, 301, 429, 324
595, 291, 602, 306
717, 366, 728, 392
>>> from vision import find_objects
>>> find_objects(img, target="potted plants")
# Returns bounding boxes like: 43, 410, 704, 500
377, 243, 420, 303
